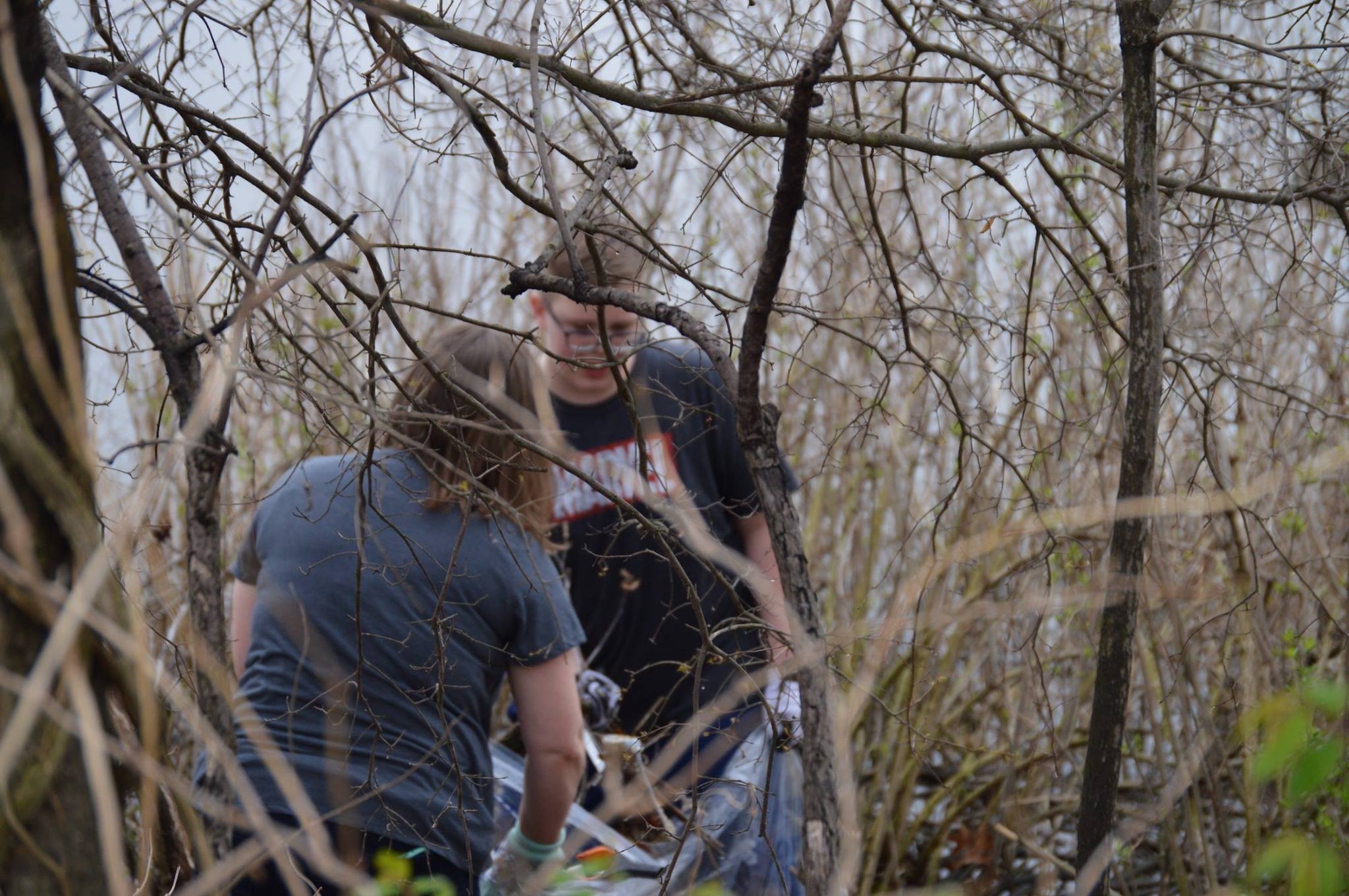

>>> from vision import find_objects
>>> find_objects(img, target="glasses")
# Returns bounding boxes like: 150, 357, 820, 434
542, 294, 645, 353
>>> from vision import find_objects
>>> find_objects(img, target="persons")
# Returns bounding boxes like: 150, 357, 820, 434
502, 216, 805, 896
188, 321, 587, 896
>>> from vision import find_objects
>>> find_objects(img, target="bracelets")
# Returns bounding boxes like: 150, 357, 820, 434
507, 820, 566, 861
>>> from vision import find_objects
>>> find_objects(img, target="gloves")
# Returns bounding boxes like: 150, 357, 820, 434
762, 680, 804, 752
482, 816, 568, 895
